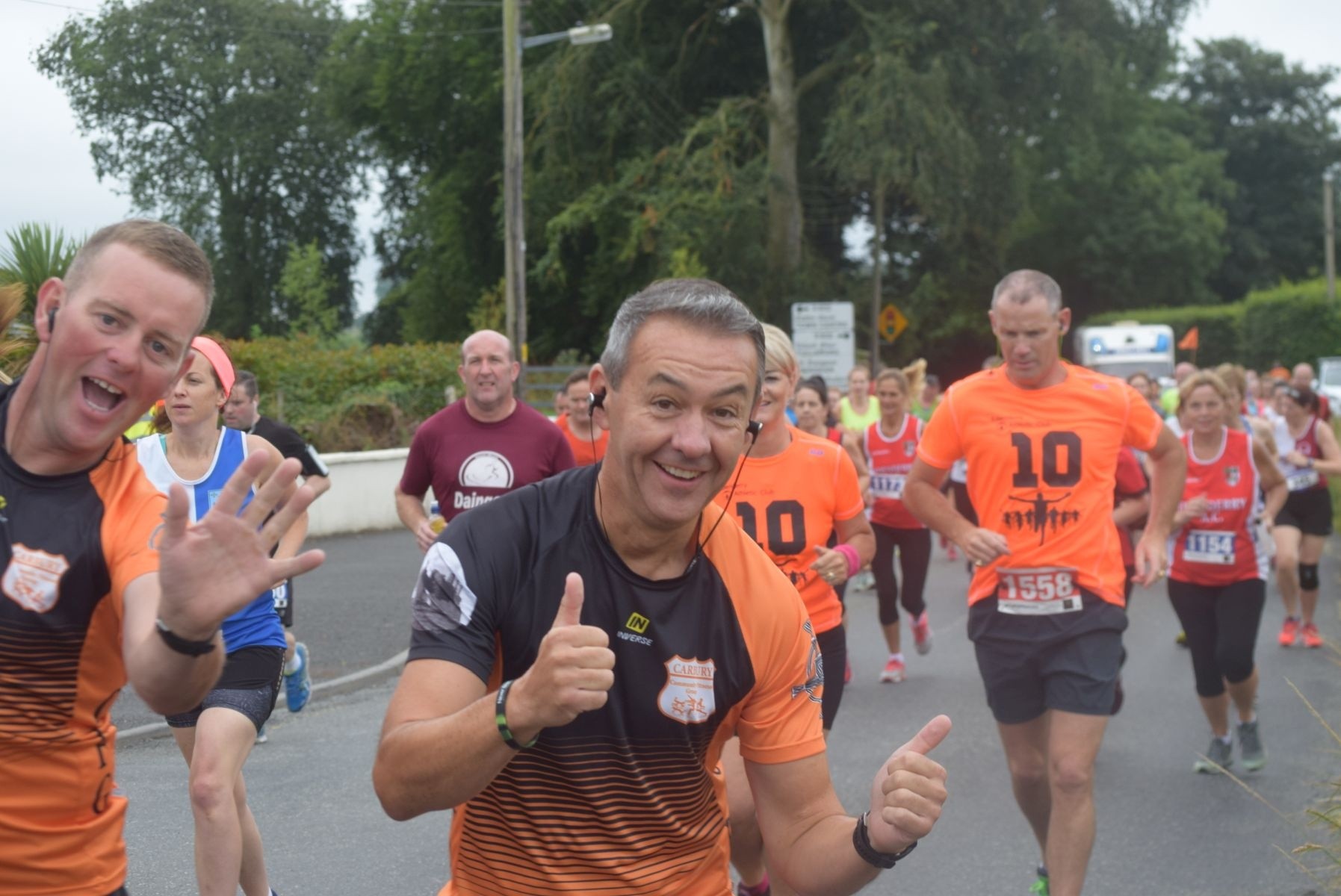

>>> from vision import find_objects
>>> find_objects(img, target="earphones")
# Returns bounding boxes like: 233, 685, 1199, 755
587, 388, 606, 417
49, 306, 59, 333
746, 420, 763, 446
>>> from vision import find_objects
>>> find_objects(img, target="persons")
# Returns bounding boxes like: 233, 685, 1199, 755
1165, 375, 1290, 775
1112, 440, 1152, 712
223, 369, 331, 744
789, 359, 1341, 647
860, 370, 951, 686
371, 274, 955, 894
554, 370, 610, 470
901, 268, 1186, 895
788, 375, 869, 684
558, 388, 572, 419
393, 329, 575, 554
0, 217, 323, 891
710, 319, 877, 896
121, 334, 314, 892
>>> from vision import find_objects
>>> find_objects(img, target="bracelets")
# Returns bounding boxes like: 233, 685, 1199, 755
156, 609, 217, 658
495, 678, 538, 750
852, 808, 917, 871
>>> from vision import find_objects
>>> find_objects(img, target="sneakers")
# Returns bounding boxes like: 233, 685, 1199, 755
1194, 731, 1234, 774
1238, 715, 1268, 770
1280, 615, 1300, 645
912, 613, 933, 654
1037, 864, 1048, 896
284, 642, 312, 712
1302, 622, 1323, 646
881, 652, 905, 682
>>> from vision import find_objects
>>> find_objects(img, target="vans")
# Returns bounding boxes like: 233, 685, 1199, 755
1072, 318, 1178, 389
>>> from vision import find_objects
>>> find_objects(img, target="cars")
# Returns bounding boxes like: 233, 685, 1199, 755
1310, 356, 1340, 418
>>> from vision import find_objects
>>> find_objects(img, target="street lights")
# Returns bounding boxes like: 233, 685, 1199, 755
502, 1, 615, 367
